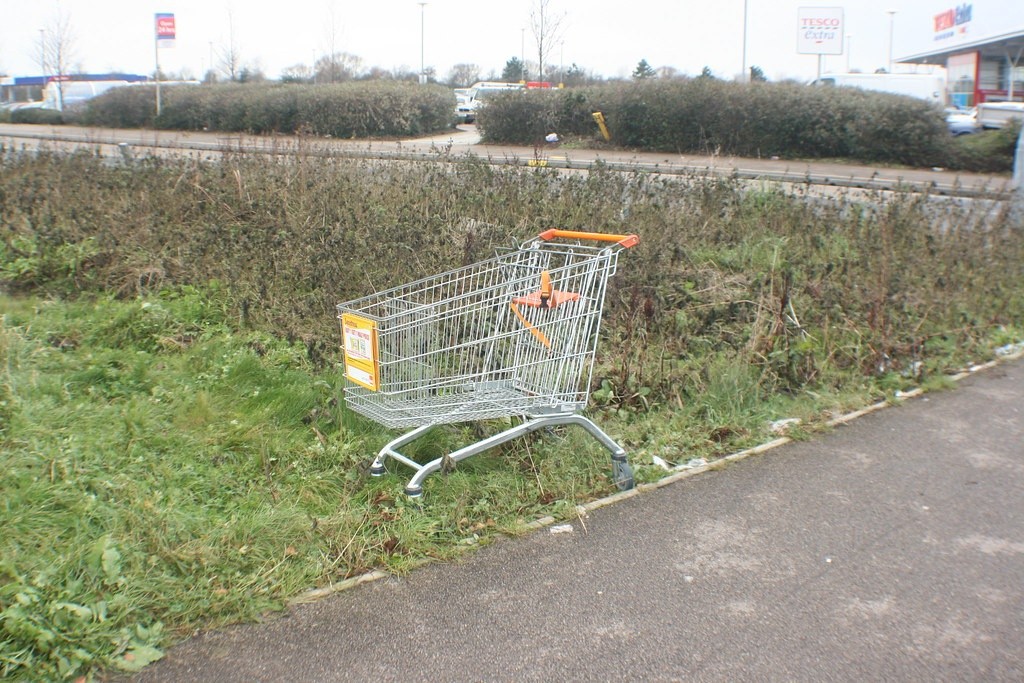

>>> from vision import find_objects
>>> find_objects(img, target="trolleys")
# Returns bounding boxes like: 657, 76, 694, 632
336, 228, 638, 510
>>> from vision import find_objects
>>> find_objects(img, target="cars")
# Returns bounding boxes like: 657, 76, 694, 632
454, 81, 560, 126
944, 106, 984, 136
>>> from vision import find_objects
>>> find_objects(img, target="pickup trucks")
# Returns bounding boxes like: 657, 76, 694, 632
976, 101, 1024, 130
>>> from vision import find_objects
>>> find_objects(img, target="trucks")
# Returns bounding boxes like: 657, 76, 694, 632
806, 73, 946, 110
